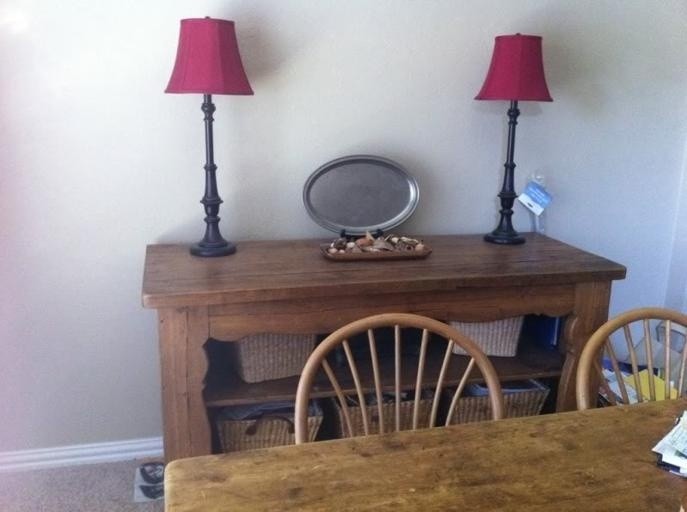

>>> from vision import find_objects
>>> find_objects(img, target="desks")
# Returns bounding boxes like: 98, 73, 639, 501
164, 395, 687, 512
140, 230, 627, 466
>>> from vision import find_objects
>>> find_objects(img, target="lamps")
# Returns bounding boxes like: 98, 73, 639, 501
474, 33, 553, 246
162, 18, 255, 259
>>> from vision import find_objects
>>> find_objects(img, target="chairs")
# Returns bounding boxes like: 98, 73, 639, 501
575, 306, 687, 413
294, 311, 507, 446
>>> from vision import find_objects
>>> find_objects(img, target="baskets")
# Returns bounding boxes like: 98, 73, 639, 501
450, 317, 523, 357
227, 334, 317, 385
330, 391, 434, 436
445, 380, 550, 424
213, 401, 322, 453
